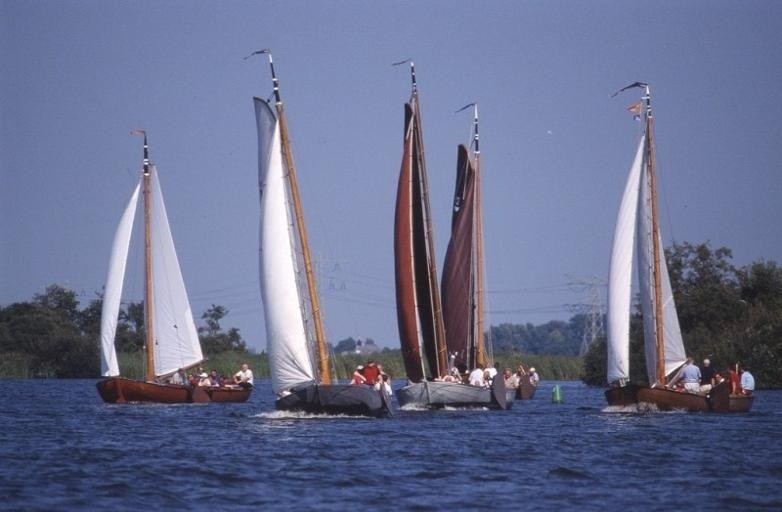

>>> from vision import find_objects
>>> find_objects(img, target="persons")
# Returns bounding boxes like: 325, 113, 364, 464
233, 362, 255, 390
678, 357, 756, 395
350, 359, 393, 397
436, 362, 541, 389
169, 365, 233, 392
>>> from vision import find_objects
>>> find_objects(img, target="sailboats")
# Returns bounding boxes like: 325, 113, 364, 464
242, 49, 397, 418
96, 130, 253, 404
604, 81, 754, 416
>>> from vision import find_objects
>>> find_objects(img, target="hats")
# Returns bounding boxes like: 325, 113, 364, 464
199, 373, 208, 377
357, 365, 364, 370
529, 367, 535, 372
368, 359, 374, 364
